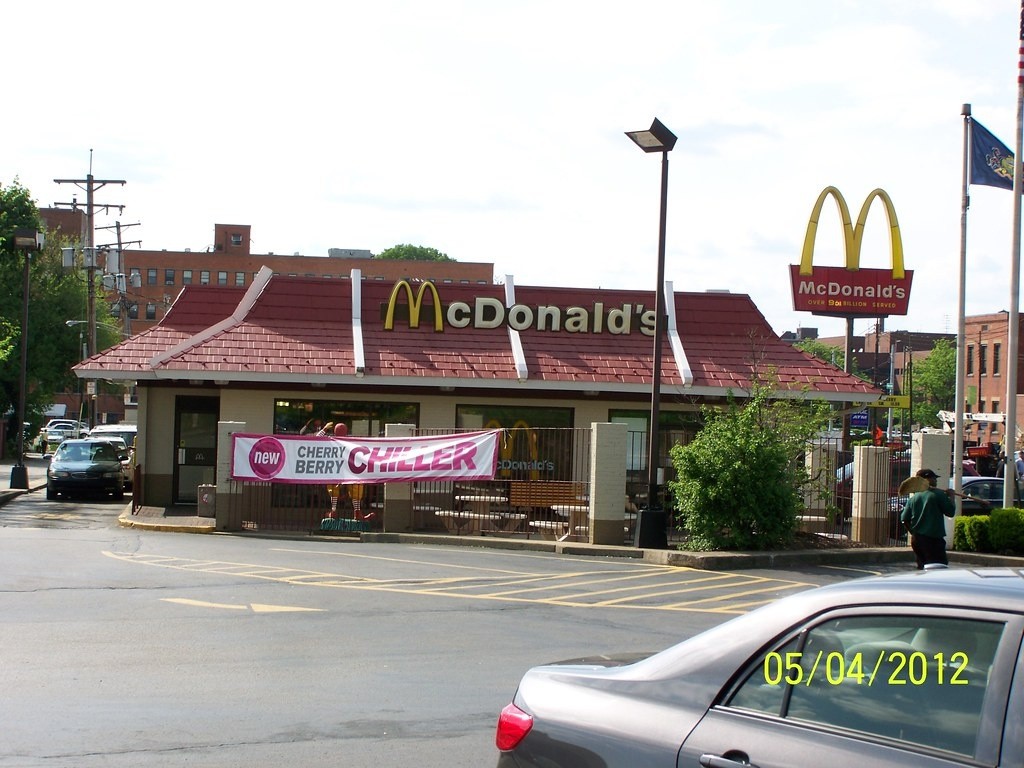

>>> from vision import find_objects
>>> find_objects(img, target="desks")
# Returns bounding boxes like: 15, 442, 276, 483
454, 496, 509, 536
551, 505, 592, 541
574, 493, 634, 503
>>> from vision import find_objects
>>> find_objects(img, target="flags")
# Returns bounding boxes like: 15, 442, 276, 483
969, 117, 1024, 195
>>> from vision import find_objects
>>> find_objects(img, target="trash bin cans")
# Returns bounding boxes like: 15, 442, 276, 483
198, 486, 216, 518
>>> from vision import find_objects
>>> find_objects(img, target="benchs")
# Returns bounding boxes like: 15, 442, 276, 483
755, 622, 990, 734
356, 503, 637, 532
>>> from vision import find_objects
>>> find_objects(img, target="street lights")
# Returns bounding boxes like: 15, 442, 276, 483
14, 236, 36, 465
623, 114, 680, 508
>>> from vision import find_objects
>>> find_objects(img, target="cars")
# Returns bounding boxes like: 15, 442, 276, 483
827, 424, 1024, 542
35, 417, 137, 494
494, 563, 1024, 768
43, 438, 128, 501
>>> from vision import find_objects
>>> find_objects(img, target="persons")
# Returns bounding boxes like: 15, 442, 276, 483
901, 469, 956, 571
996, 449, 1024, 479
299, 418, 321, 435
316, 422, 376, 519
40, 429, 48, 456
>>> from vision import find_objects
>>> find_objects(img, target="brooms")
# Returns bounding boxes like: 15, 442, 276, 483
899, 476, 991, 506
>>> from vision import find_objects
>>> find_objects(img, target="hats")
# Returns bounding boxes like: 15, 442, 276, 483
917, 469, 941, 479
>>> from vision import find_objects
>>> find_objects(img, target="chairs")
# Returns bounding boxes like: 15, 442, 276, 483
92, 448, 110, 460
